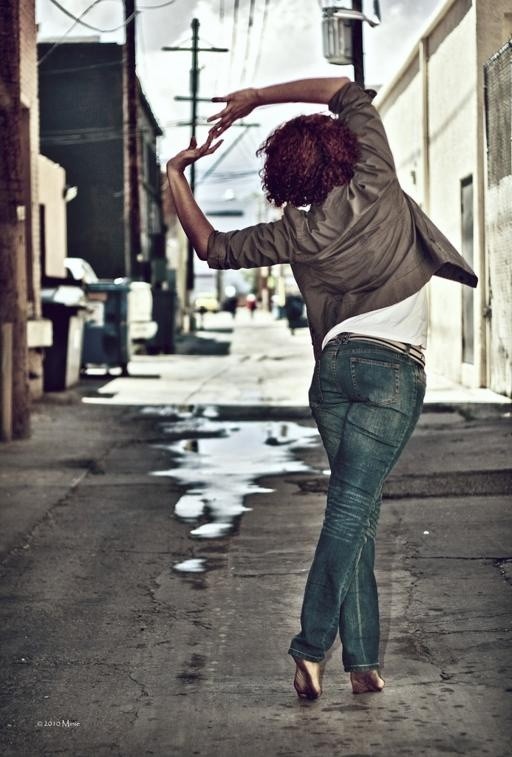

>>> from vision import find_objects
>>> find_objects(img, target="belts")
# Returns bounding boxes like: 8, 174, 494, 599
330, 332, 425, 368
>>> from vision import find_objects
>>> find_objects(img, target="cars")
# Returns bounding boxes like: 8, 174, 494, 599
65, 256, 160, 341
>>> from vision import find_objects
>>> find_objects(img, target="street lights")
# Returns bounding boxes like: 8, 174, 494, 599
223, 188, 274, 313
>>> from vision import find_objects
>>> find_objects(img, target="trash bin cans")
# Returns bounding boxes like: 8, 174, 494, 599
42, 295, 88, 391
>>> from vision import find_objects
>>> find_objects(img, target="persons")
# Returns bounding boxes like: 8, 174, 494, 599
165, 75, 477, 700
